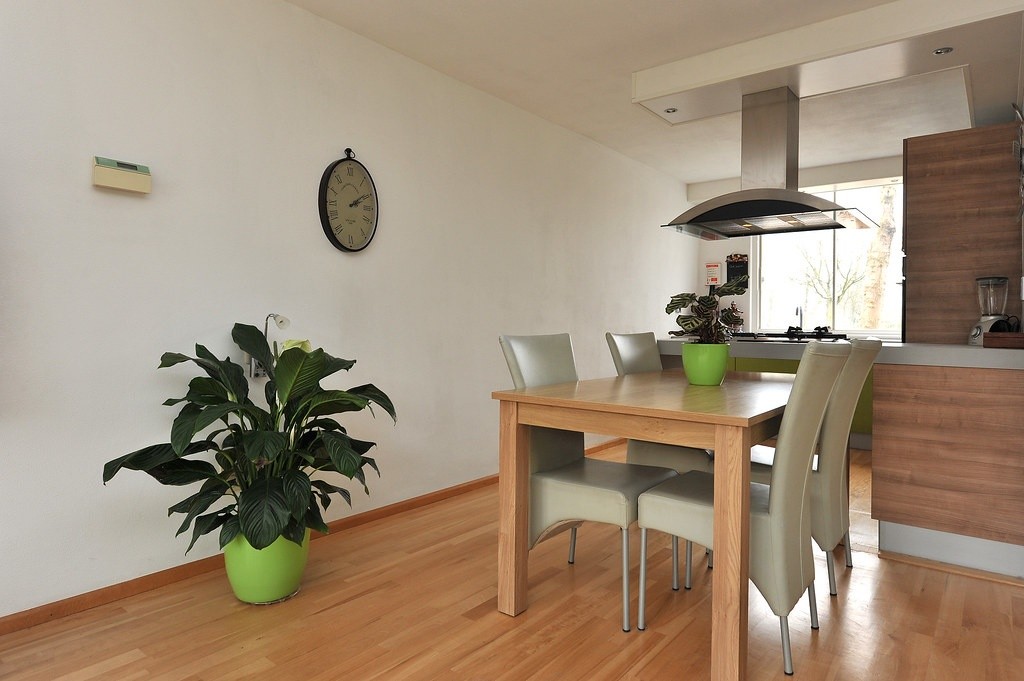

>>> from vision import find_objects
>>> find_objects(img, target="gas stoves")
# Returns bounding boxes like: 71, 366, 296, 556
729, 326, 849, 343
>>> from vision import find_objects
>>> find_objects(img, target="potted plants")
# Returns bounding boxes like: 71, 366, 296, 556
666, 274, 750, 386
103, 323, 397, 604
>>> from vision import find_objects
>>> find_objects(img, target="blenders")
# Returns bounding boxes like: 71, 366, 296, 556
967, 277, 1013, 345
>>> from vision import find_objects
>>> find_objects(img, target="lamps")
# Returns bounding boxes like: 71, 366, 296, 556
250, 314, 291, 379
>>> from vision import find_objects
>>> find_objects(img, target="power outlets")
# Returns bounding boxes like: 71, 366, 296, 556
251, 358, 267, 377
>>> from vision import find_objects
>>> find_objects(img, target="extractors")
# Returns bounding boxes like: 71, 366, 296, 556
659, 144, 880, 242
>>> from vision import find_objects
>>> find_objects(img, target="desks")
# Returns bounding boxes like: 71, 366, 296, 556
492, 367, 795, 681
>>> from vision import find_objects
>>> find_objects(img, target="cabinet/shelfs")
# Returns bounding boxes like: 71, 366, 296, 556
901, 121, 1024, 343
660, 353, 873, 451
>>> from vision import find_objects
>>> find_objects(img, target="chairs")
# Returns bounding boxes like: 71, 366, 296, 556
497, 333, 677, 633
708, 339, 882, 596
638, 341, 849, 676
605, 331, 713, 590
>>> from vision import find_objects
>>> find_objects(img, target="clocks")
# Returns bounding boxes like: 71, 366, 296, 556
319, 148, 379, 253
